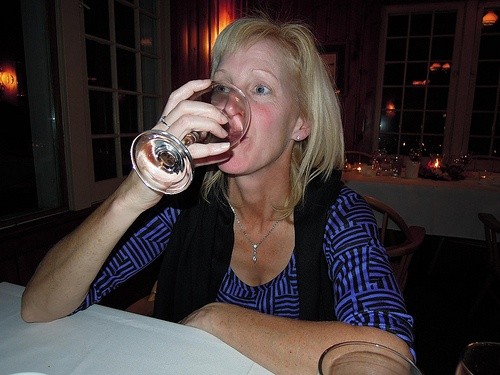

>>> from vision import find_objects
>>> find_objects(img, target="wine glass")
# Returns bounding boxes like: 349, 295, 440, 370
129, 82, 251, 194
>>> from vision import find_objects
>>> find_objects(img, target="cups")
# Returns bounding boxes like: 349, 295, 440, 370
454, 341, 500, 375
343, 148, 489, 180
317, 341, 423, 375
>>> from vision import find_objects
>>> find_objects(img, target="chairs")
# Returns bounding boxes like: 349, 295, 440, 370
466, 213, 500, 320
364, 197, 425, 290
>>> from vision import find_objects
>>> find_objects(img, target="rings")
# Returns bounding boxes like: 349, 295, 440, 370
161, 119, 170, 128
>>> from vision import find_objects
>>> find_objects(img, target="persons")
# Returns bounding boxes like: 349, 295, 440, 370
19, 8, 416, 375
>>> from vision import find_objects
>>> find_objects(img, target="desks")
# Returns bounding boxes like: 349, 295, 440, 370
1, 281, 276, 375
343, 172, 500, 241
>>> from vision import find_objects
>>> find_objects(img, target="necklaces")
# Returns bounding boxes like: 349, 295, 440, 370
226, 197, 280, 264
